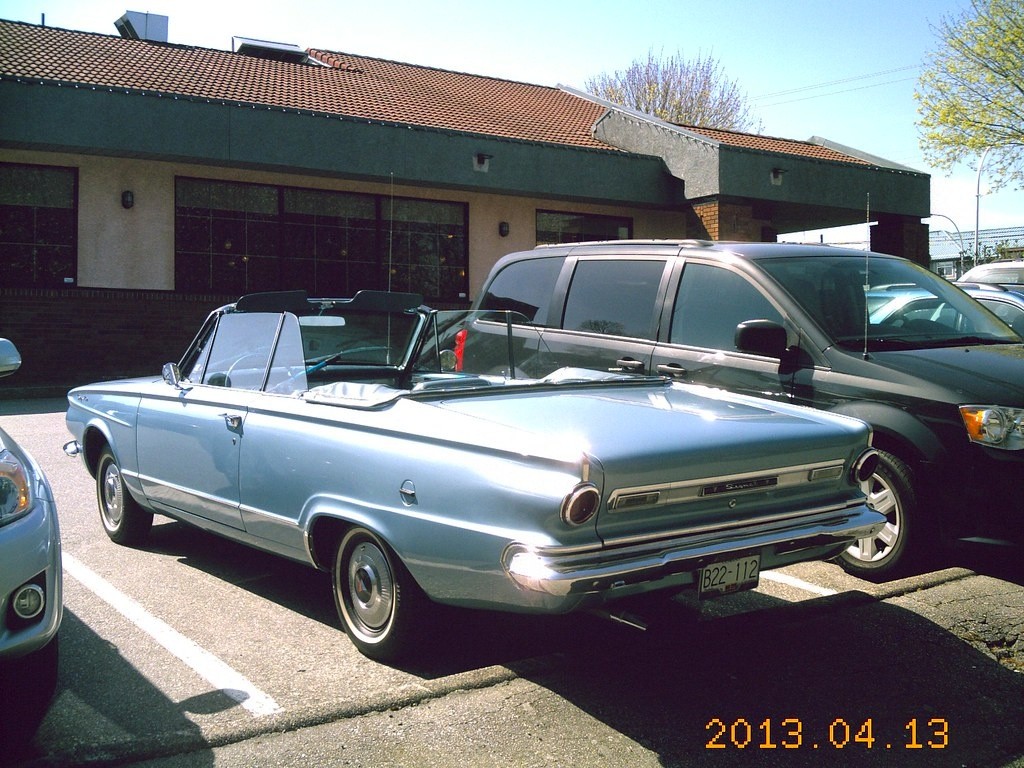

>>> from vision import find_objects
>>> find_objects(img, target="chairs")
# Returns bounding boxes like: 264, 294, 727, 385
767, 283, 828, 347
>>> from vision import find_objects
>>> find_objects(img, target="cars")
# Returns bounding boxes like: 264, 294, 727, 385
864, 260, 1024, 343
0, 338, 63, 717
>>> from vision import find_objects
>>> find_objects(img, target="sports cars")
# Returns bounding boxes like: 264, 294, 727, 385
65, 287, 887, 664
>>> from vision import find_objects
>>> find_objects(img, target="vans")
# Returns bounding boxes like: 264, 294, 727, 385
467, 238, 1024, 584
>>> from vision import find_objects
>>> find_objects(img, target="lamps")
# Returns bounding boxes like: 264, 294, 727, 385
477, 152, 494, 164
499, 221, 509, 237
772, 168, 788, 179
121, 190, 134, 209
